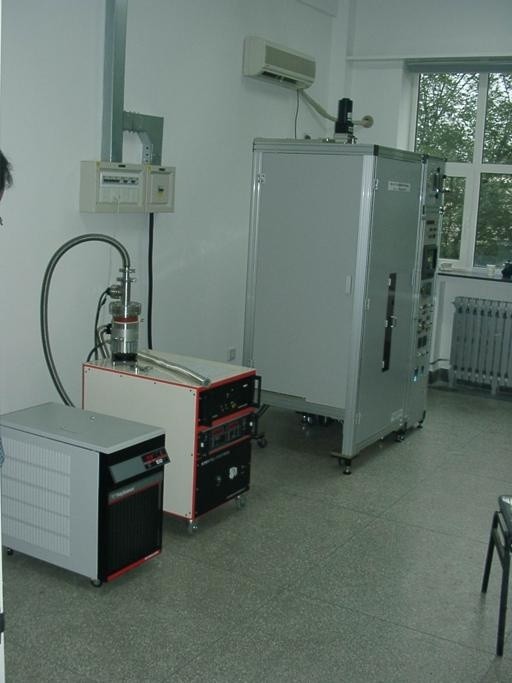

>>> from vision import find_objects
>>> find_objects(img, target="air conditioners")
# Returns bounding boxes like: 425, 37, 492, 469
243, 36, 317, 93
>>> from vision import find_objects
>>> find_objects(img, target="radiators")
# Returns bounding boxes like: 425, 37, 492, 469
446, 296, 511, 395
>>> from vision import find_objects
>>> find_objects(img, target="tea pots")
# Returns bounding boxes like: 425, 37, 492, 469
501, 260, 512, 279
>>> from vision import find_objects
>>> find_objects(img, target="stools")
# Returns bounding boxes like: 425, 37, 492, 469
481, 494, 512, 660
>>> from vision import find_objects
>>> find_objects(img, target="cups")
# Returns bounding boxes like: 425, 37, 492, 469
440, 262, 452, 270
486, 264, 496, 277
